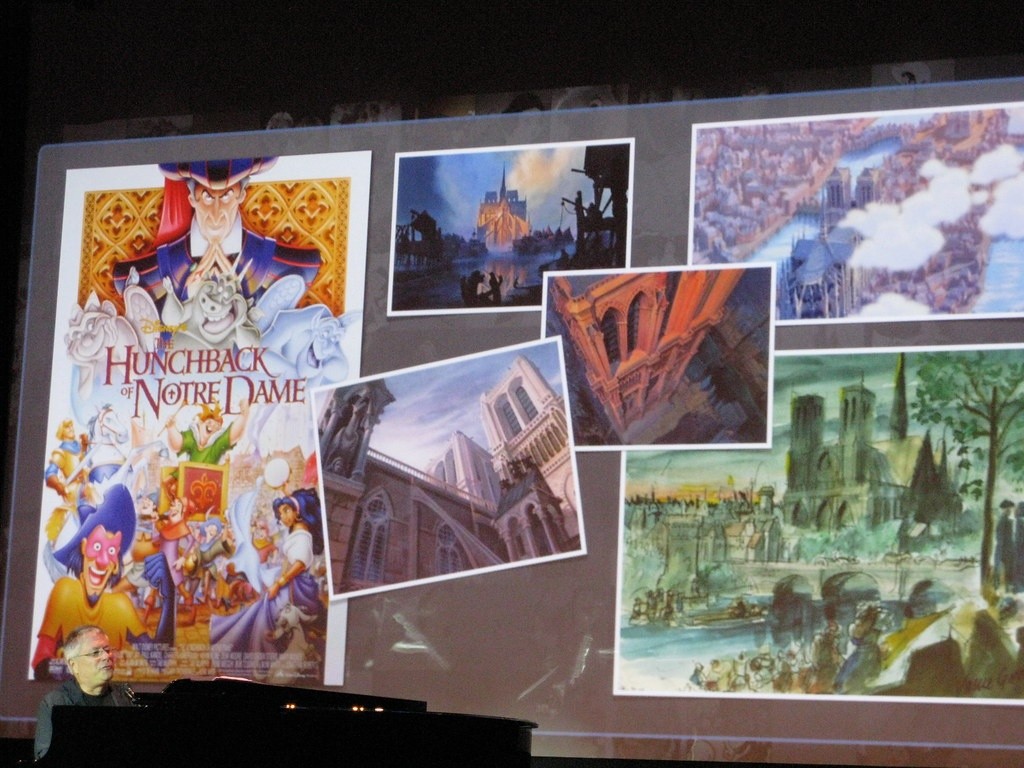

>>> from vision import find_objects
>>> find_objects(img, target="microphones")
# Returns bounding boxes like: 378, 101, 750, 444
118, 682, 142, 706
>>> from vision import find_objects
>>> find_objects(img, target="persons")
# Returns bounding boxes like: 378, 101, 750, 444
33, 626, 135, 762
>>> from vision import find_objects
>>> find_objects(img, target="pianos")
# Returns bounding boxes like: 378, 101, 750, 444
7, 674, 538, 768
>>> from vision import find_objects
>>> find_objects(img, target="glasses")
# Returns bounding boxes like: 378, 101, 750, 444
72, 648, 112, 658
